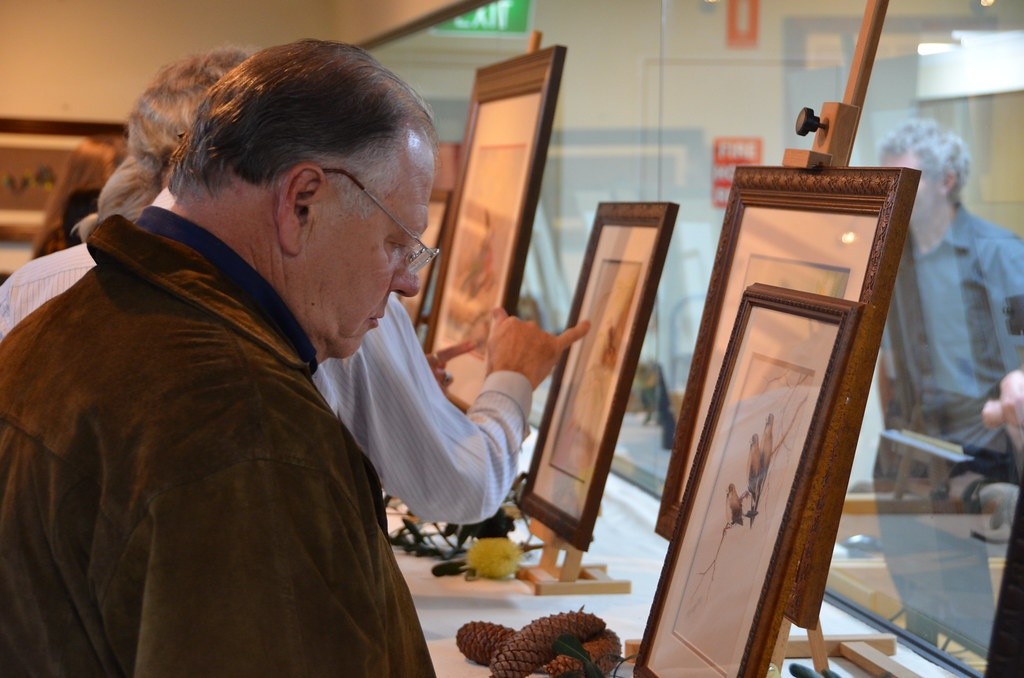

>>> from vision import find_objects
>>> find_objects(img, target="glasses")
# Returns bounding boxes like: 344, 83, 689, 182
324, 169, 440, 275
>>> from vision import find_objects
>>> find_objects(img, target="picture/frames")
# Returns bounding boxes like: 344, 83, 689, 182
632, 281, 865, 678
400, 187, 453, 331
519, 203, 681, 551
651, 165, 922, 631
422, 44, 569, 412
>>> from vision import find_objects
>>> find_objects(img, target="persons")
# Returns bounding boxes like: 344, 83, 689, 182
0, 34, 440, 678
871, 119, 1024, 648
0, 44, 593, 522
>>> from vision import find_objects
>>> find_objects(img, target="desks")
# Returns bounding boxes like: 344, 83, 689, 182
386, 422, 966, 678
